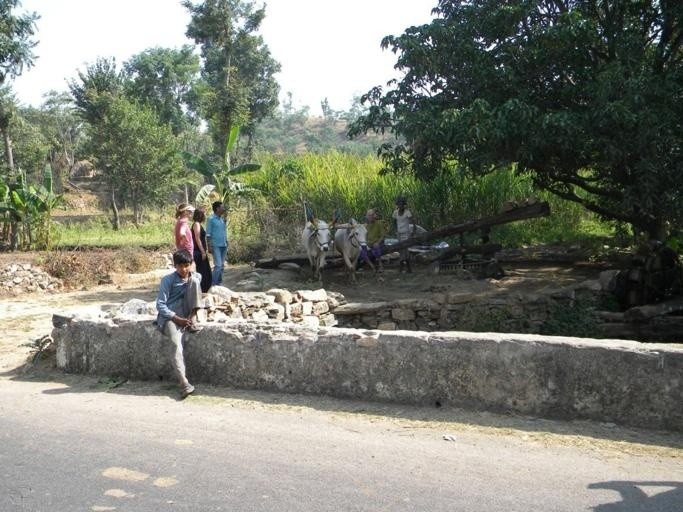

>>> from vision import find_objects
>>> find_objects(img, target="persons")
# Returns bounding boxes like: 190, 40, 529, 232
188, 257, 202, 296
154, 249, 204, 399
173, 202, 195, 258
204, 200, 229, 286
189, 208, 212, 293
386, 199, 415, 275
358, 208, 387, 274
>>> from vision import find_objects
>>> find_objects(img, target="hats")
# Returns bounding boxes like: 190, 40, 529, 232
179, 204, 195, 211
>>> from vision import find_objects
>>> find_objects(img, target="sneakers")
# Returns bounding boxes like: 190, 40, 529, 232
188, 320, 199, 333
181, 381, 195, 399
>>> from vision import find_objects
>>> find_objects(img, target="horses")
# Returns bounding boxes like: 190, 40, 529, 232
334, 218, 367, 281
302, 218, 333, 281
